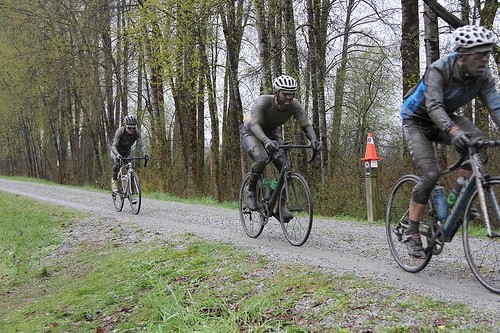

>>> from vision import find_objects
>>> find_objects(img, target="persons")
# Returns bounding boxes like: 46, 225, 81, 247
111, 116, 148, 193
240, 75, 321, 220
399, 24, 500, 259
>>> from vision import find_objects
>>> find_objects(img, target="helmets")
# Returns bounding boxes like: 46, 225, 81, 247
124, 116, 137, 128
451, 25, 498, 54
272, 74, 298, 93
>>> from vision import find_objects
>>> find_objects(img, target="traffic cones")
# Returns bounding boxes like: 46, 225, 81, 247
359, 132, 380, 161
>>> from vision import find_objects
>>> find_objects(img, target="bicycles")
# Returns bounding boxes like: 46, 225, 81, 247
110, 155, 148, 215
385, 137, 500, 295
237, 141, 322, 246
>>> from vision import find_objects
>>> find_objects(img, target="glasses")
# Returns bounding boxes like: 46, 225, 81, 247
128, 127, 136, 130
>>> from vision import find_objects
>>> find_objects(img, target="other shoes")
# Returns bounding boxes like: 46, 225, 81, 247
401, 230, 423, 258
276, 207, 293, 220
129, 196, 137, 203
244, 184, 257, 210
469, 204, 481, 220
111, 177, 118, 191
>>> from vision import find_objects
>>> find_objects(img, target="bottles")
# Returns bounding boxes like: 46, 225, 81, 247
122, 174, 127, 188
262, 176, 270, 196
432, 184, 450, 219
447, 177, 466, 208
265, 179, 278, 200
